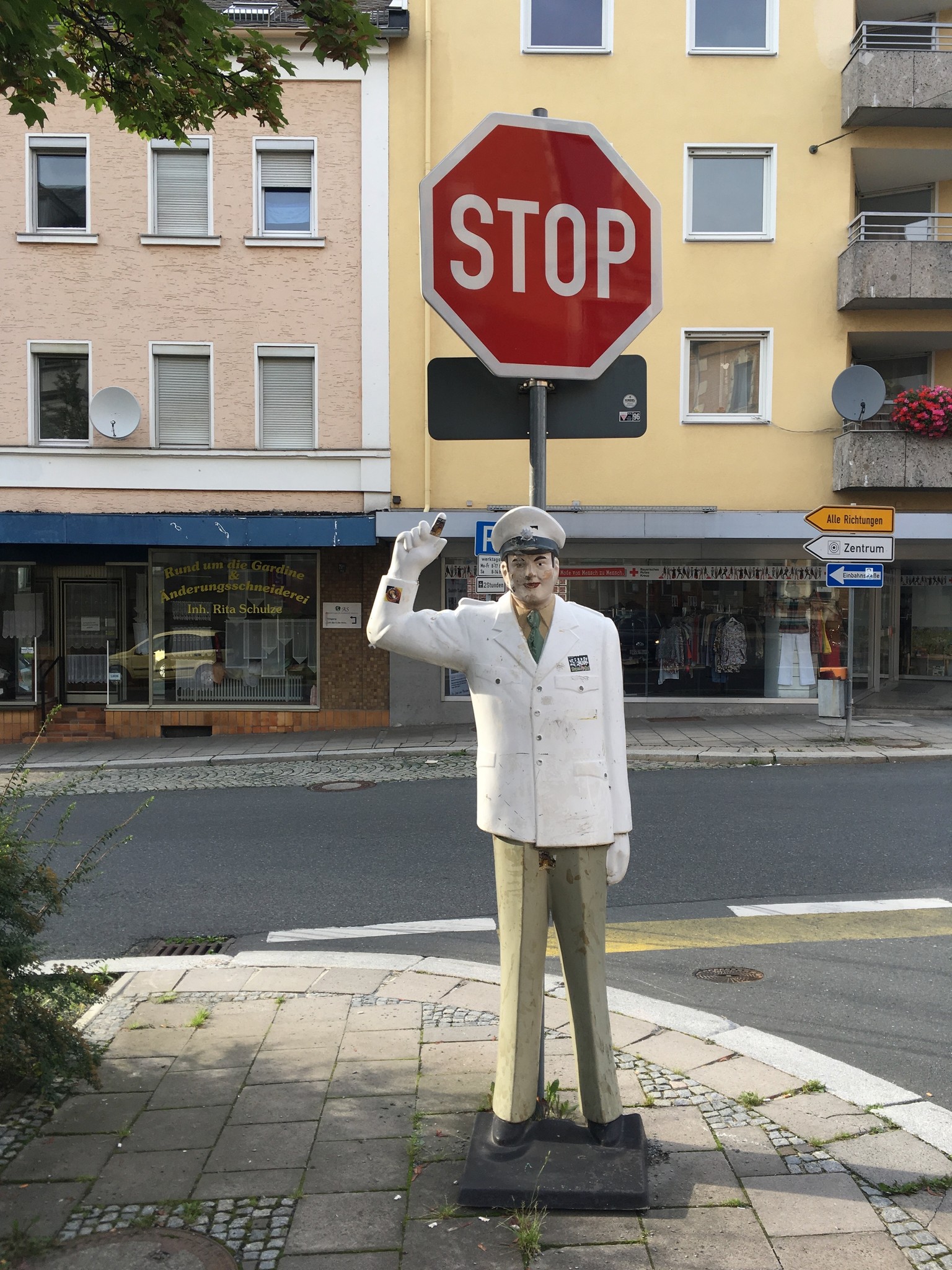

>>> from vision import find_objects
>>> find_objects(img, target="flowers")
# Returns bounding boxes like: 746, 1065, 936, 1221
890, 383, 952, 439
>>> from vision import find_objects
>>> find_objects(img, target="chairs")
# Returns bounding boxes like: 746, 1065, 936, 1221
901, 652, 918, 675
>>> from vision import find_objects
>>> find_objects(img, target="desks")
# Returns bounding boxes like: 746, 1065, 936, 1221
909, 654, 946, 677
944, 655, 952, 677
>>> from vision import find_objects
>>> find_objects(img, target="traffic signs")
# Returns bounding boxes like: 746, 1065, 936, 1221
804, 504, 895, 588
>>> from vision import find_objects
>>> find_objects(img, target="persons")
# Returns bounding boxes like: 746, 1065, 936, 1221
821, 587, 844, 668
367, 501, 640, 1153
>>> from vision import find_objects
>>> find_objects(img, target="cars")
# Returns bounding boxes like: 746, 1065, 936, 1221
617, 613, 671, 664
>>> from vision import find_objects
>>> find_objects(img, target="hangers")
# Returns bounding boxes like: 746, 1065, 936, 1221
671, 610, 697, 624
785, 581, 804, 596
714, 611, 726, 621
726, 612, 739, 624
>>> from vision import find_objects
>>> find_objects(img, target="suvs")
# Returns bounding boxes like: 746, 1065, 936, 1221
109, 630, 234, 691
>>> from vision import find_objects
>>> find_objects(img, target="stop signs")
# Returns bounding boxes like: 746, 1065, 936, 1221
418, 113, 662, 379
